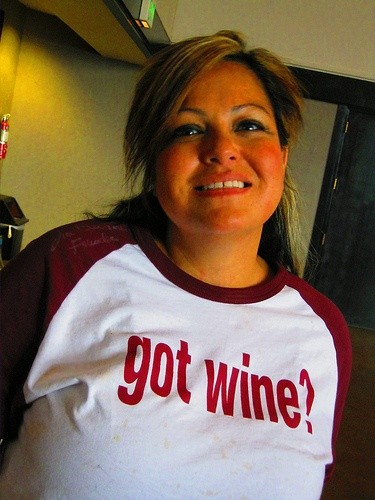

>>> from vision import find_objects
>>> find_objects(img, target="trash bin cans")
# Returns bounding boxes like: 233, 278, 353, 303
0, 194, 29, 261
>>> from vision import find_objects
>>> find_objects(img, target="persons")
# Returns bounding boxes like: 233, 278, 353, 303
0, 30, 354, 500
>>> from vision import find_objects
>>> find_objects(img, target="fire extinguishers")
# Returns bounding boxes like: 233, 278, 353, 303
0, 114, 12, 160
